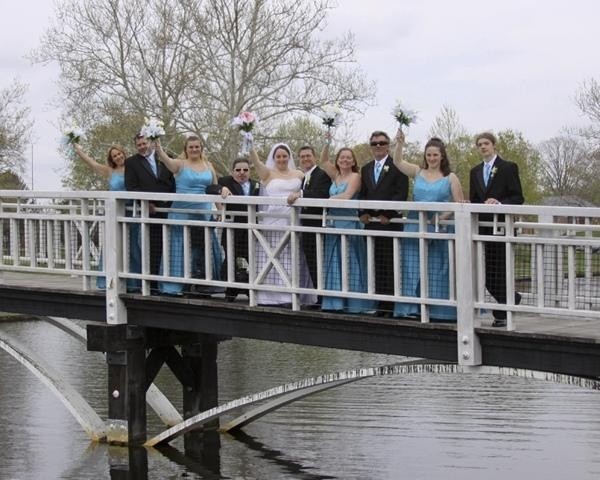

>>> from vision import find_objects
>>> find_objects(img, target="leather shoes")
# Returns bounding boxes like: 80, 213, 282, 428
373, 311, 385, 317
492, 318, 506, 327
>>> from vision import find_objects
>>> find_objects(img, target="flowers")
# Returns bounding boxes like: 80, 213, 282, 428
392, 103, 417, 131
306, 175, 311, 184
318, 104, 339, 131
139, 119, 165, 141
255, 183, 260, 189
233, 111, 257, 143
66, 124, 88, 145
489, 165, 498, 178
383, 165, 390, 173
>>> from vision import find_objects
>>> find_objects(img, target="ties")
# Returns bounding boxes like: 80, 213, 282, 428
484, 164, 490, 188
375, 163, 381, 184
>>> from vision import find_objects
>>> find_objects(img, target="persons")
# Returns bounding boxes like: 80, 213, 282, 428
154, 137, 217, 294
244, 133, 317, 306
125, 134, 176, 293
206, 158, 260, 303
358, 132, 408, 317
322, 148, 367, 314
469, 133, 524, 328
73, 140, 141, 292
393, 127, 464, 322
287, 147, 331, 310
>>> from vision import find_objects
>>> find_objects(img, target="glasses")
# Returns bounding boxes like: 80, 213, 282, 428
235, 168, 249, 172
370, 141, 388, 146
